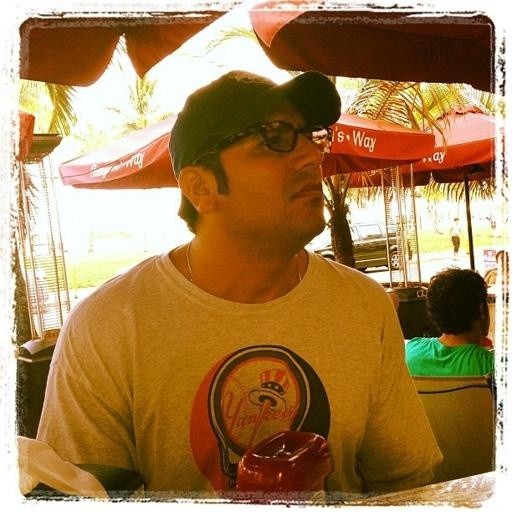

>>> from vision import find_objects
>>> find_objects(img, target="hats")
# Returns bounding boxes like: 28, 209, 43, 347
170, 71, 341, 180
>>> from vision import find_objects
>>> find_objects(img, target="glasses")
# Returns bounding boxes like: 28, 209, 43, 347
192, 120, 331, 165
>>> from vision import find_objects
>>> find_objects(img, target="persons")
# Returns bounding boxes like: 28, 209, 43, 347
404, 266, 495, 378
36, 70, 444, 500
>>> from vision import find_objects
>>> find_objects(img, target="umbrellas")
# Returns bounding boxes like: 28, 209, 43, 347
58, 111, 436, 189
334, 103, 505, 271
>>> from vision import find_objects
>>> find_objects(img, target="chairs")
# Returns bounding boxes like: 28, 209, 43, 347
410, 374, 496, 484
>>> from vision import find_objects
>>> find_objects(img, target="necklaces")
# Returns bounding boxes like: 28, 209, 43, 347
186, 238, 302, 283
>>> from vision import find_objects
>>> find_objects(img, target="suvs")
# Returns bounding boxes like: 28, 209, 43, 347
305, 217, 413, 273
